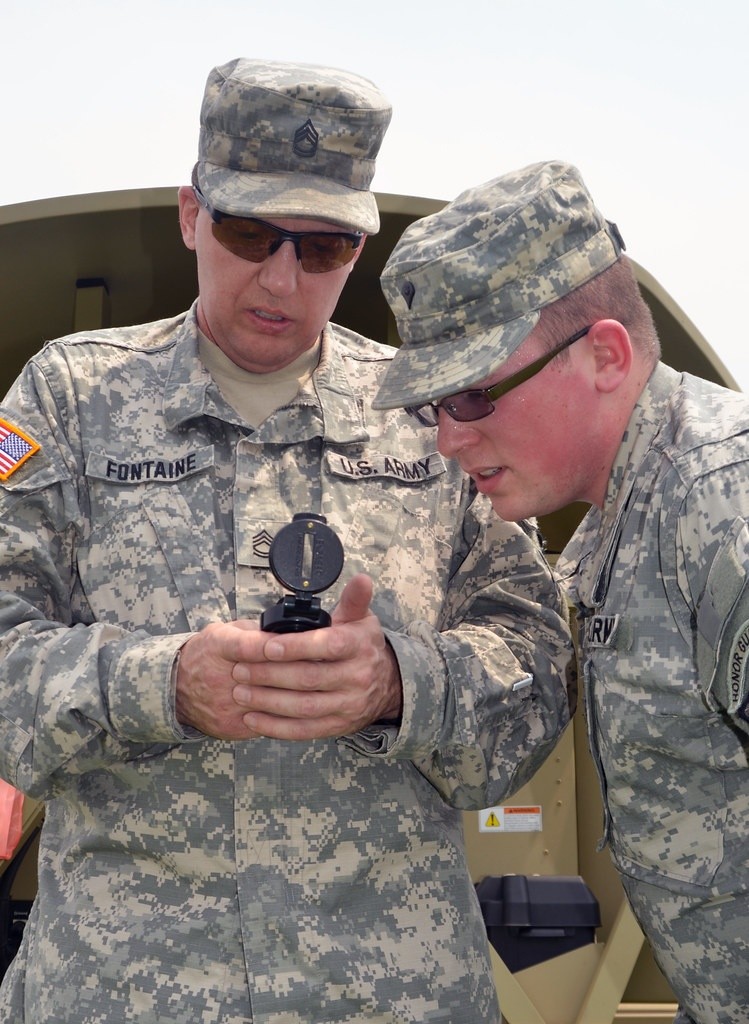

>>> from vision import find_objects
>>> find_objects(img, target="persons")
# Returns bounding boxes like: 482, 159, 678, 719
369, 162, 749, 1024
1, 56, 579, 1024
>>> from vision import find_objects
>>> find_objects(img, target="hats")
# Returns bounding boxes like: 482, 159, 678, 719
370, 161, 627, 411
198, 57, 393, 235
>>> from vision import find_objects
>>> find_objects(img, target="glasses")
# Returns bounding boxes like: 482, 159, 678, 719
192, 185, 364, 273
406, 326, 595, 427
